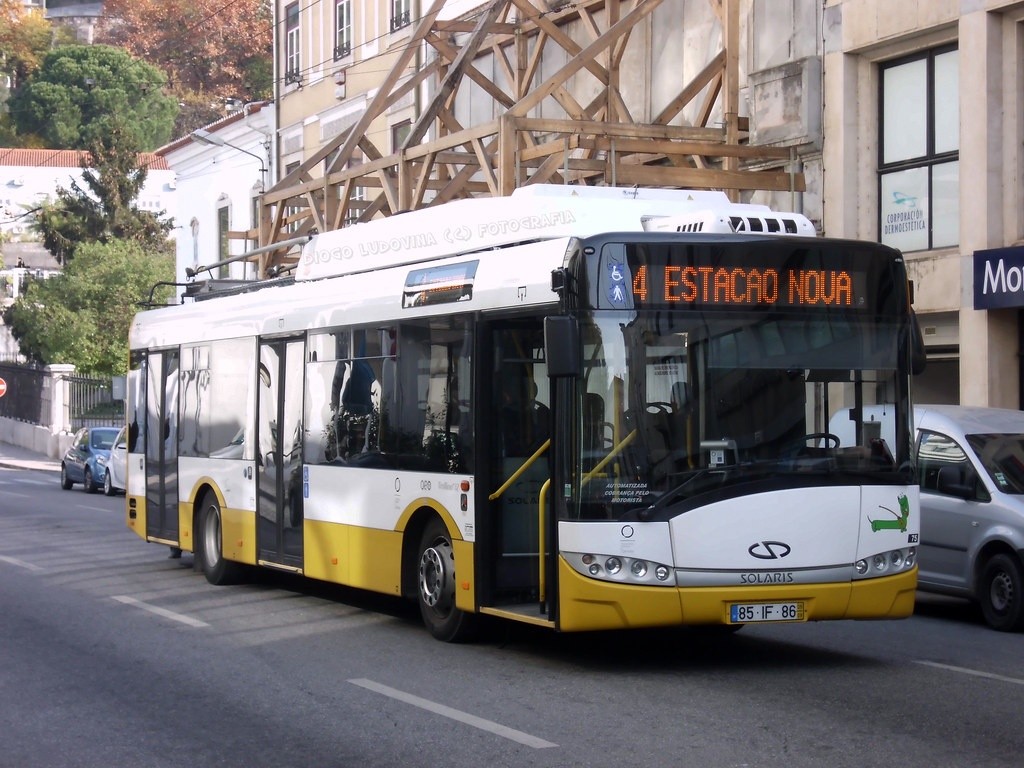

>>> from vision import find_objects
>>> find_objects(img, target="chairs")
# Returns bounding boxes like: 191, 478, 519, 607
625, 402, 679, 492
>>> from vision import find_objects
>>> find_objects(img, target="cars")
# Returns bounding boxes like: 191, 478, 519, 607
104, 424, 130, 497
60, 425, 122, 494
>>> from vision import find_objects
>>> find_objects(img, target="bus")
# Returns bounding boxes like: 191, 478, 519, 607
119, 183, 929, 645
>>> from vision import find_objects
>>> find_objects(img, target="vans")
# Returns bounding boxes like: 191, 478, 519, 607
818, 403, 1024, 636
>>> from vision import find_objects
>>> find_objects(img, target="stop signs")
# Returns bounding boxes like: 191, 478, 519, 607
0, 377, 8, 399
